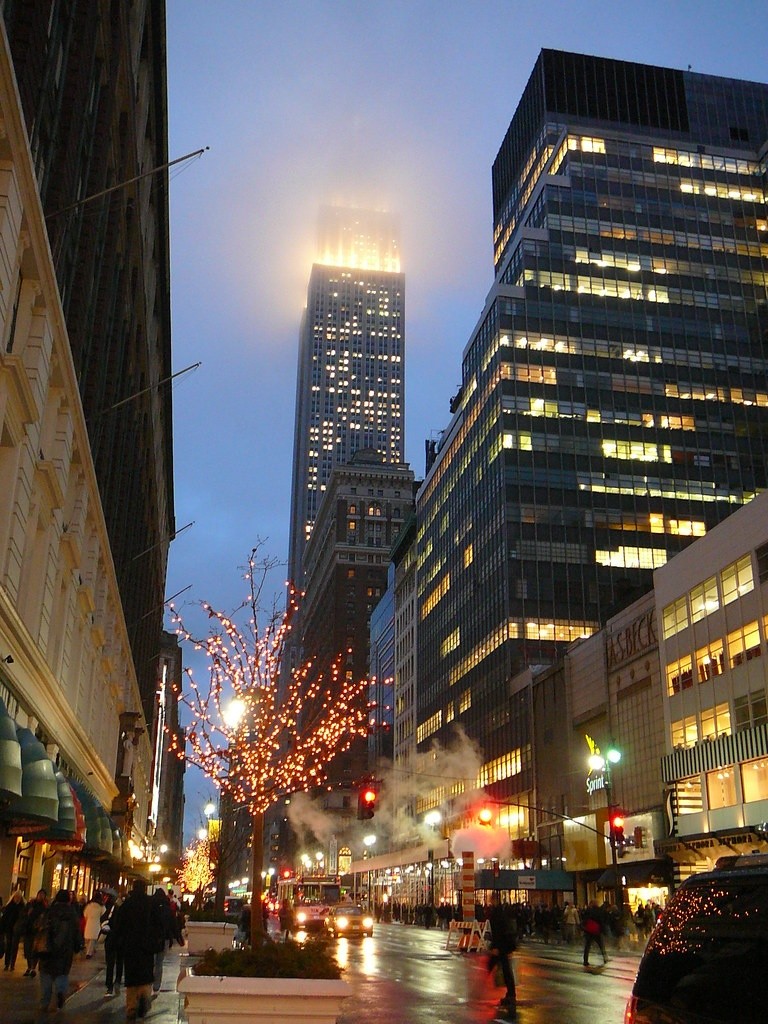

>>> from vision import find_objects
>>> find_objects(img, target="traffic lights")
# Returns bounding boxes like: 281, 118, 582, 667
612, 815, 625, 842
478, 807, 493, 825
356, 789, 377, 821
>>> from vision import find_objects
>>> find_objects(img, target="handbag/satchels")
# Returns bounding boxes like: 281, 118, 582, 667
495, 958, 517, 986
100, 920, 111, 934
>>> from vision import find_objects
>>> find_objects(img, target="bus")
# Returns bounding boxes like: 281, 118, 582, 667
276, 875, 342, 931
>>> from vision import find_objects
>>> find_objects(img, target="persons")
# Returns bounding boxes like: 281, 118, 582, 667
0, 879, 190, 1024
375, 899, 664, 951
582, 899, 613, 966
278, 898, 293, 944
488, 891, 519, 1024
203, 900, 215, 913
238, 905, 251, 946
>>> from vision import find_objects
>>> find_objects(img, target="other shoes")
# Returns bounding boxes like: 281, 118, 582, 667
23, 969, 29, 976
56, 999, 63, 1009
153, 987, 160, 995
29, 969, 36, 977
115, 981, 120, 994
138, 997, 147, 1017
10, 965, 14, 971
41, 997, 50, 1004
104, 991, 113, 996
4, 967, 9, 970
126, 1009, 138, 1022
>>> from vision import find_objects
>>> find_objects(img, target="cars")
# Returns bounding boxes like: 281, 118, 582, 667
323, 903, 374, 938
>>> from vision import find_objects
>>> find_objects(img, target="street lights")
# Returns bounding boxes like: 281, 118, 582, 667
214, 699, 249, 923
587, 746, 624, 936
149, 863, 162, 896
364, 834, 377, 913
423, 811, 441, 927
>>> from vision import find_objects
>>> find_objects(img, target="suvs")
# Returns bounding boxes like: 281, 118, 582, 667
622, 852, 768, 1024
